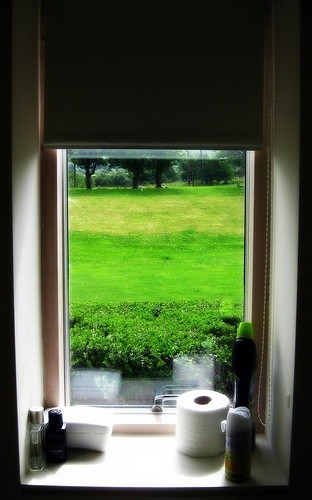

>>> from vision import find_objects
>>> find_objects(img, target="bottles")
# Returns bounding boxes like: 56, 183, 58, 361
28, 405, 67, 472
224, 407, 253, 481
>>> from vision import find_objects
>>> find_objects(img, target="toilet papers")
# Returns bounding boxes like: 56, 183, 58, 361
173, 354, 215, 394
173, 390, 230, 459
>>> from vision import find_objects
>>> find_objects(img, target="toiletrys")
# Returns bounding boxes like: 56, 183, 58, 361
28, 406, 46, 472
230, 321, 257, 451
224, 407, 254, 483
45, 408, 67, 463
